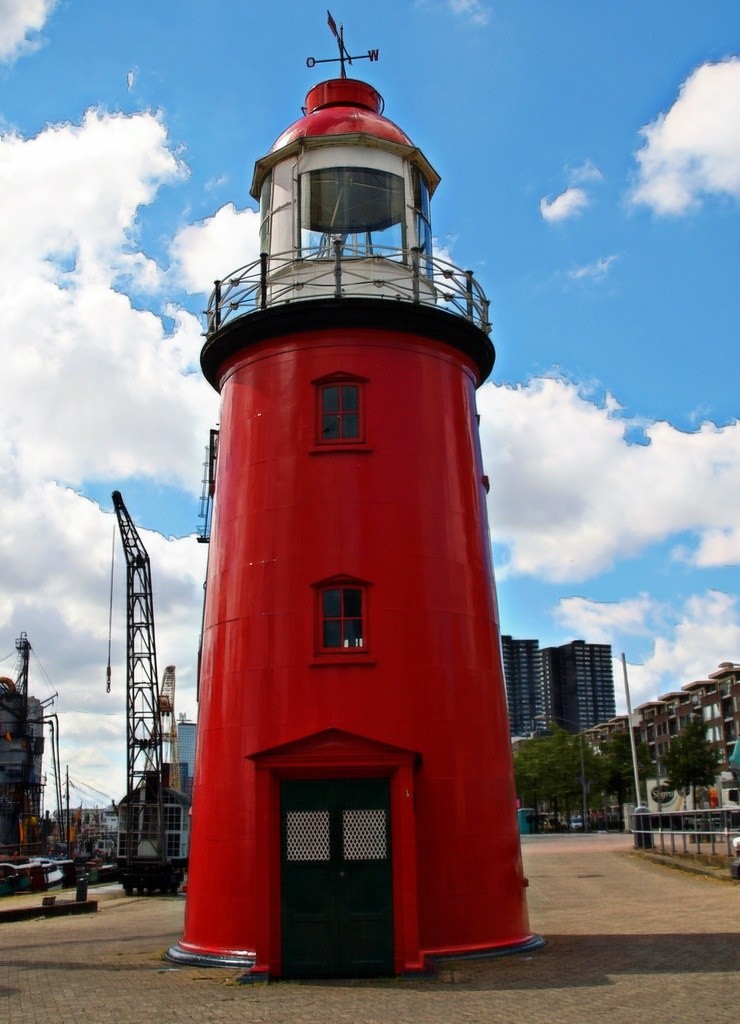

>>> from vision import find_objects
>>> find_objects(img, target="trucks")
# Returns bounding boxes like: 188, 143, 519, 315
646, 773, 740, 828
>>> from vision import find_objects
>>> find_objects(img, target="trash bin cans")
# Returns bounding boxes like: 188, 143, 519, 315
518, 807, 535, 833
634, 807, 653, 848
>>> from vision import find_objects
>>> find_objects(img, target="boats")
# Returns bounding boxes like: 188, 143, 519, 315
0, 630, 115, 895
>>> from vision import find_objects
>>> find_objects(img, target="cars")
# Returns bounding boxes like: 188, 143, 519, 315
538, 812, 592, 832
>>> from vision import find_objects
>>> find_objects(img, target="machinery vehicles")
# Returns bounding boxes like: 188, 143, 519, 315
103, 491, 189, 896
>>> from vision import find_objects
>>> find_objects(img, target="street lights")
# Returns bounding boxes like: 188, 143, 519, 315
533, 714, 590, 832
